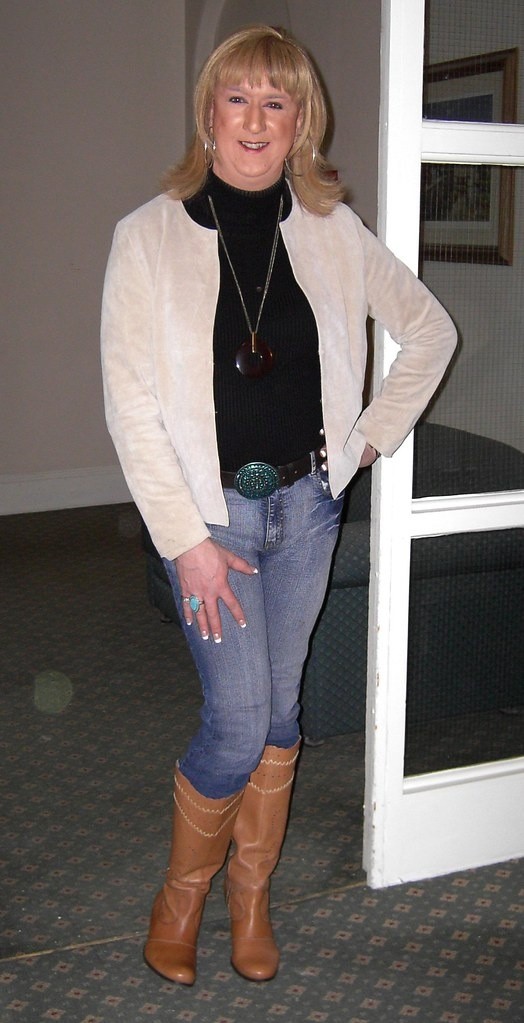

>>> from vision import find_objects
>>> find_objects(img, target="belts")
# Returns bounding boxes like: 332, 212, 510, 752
227, 449, 327, 500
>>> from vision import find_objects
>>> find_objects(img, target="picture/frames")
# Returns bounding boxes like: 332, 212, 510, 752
422, 46, 519, 267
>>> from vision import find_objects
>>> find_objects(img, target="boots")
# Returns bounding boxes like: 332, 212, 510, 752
223, 735, 302, 981
145, 758, 246, 986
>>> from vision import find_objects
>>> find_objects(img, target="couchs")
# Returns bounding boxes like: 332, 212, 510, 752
140, 421, 524, 745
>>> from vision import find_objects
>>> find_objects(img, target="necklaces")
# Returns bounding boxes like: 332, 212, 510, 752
209, 194, 290, 377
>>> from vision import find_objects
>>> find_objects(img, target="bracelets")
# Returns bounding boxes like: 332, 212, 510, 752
370, 445, 380, 458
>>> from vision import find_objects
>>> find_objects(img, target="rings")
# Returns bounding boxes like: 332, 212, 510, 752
182, 595, 204, 613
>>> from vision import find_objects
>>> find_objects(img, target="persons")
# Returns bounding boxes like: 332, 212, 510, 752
100, 25, 458, 984
